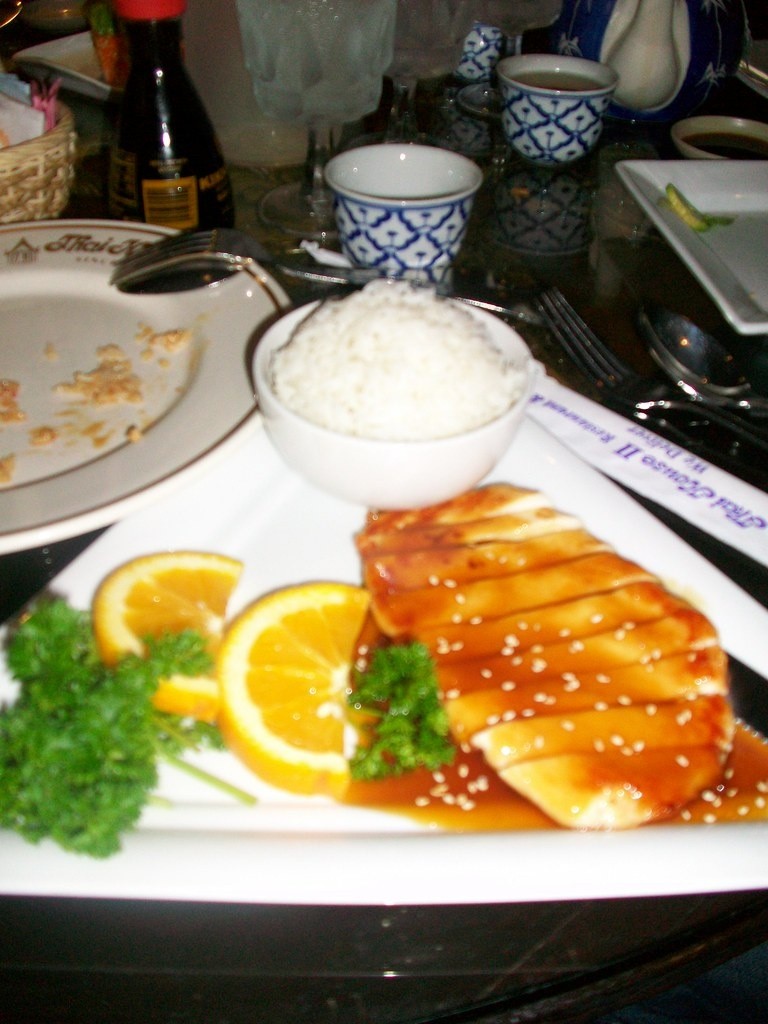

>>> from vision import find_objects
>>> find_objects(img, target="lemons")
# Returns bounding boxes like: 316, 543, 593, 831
666, 184, 710, 233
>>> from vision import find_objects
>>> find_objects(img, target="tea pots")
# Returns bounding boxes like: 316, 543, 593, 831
556, 0, 721, 126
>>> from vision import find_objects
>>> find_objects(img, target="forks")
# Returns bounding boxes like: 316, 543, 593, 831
113, 230, 610, 341
536, 281, 768, 465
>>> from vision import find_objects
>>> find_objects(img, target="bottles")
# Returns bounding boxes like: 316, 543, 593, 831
107, 0, 234, 228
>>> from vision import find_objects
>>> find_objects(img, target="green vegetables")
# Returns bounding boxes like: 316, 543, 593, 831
0, 598, 261, 858
343, 639, 460, 782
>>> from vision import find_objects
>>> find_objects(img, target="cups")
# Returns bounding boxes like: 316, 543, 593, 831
20, 0, 89, 34
451, 21, 507, 82
494, 155, 594, 256
429, 84, 503, 160
177, 0, 342, 167
497, 54, 620, 167
325, 144, 483, 263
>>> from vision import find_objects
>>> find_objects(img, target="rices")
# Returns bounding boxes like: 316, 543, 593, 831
267, 279, 526, 441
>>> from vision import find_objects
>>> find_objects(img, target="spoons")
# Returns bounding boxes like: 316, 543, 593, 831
641, 305, 768, 422
119, 265, 249, 293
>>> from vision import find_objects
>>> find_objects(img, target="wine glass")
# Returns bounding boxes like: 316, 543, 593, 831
237, 0, 397, 240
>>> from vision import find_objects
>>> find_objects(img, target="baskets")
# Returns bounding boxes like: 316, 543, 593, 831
0, 100, 75, 224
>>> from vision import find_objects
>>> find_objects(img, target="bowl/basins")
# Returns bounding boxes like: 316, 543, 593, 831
253, 302, 543, 516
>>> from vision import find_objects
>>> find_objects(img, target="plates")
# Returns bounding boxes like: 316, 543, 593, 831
616, 160, 768, 336
0, 0, 23, 29
0, 219, 292, 555
670, 115, 768, 159
12, 32, 127, 107
0, 381, 768, 903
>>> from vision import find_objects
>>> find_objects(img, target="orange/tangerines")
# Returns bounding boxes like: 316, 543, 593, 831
95, 549, 244, 720
216, 579, 369, 796
